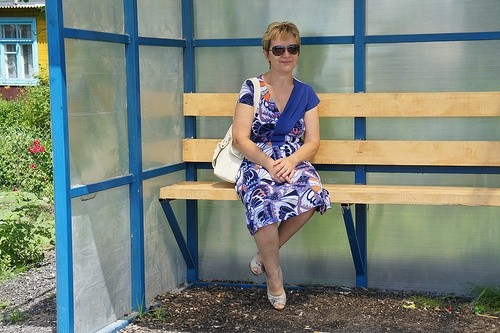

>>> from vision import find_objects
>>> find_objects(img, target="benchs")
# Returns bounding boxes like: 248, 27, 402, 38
159, 92, 500, 288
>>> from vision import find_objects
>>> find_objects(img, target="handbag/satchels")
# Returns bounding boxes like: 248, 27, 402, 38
211, 124, 244, 183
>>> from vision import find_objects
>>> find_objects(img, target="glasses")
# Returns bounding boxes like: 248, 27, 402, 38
268, 44, 299, 56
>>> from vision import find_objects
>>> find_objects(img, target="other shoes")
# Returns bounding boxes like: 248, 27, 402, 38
266, 267, 286, 310
249, 252, 266, 276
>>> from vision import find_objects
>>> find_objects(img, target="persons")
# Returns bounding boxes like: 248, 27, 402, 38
232, 22, 331, 310
